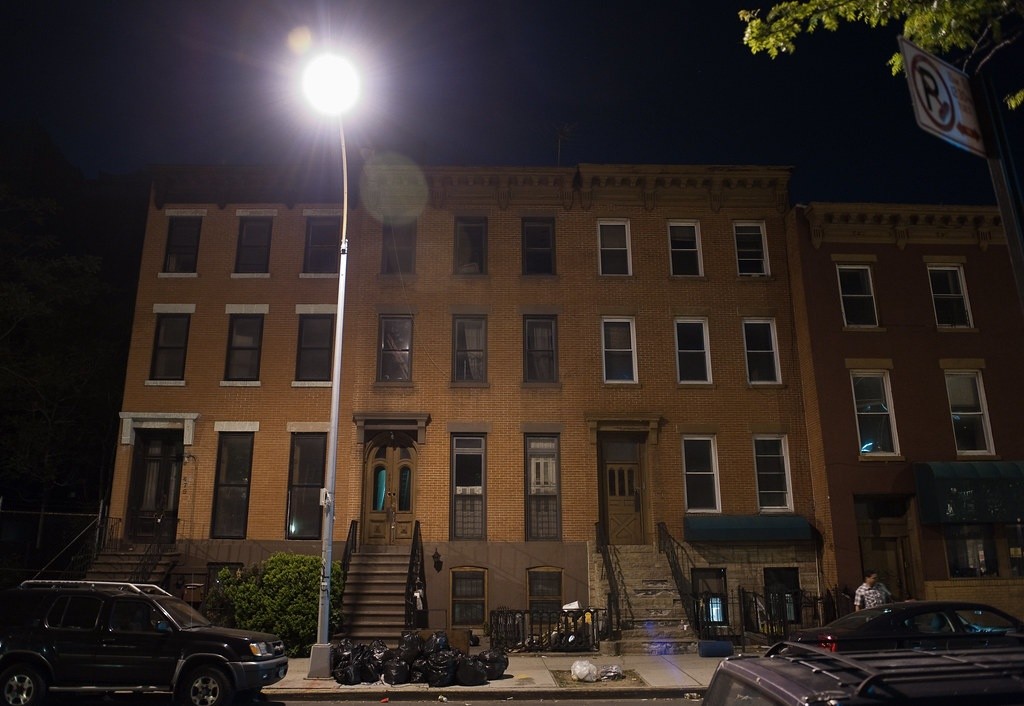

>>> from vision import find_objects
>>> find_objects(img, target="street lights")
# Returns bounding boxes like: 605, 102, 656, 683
298, 51, 358, 680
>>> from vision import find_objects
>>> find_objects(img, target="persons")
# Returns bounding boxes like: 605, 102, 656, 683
854, 570, 895, 611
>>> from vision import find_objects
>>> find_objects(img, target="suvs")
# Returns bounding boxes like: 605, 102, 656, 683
698, 633, 1024, 706
791, 599, 1024, 651
0, 578, 289, 706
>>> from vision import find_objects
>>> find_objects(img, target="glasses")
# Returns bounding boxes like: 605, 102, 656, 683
869, 577, 879, 579
